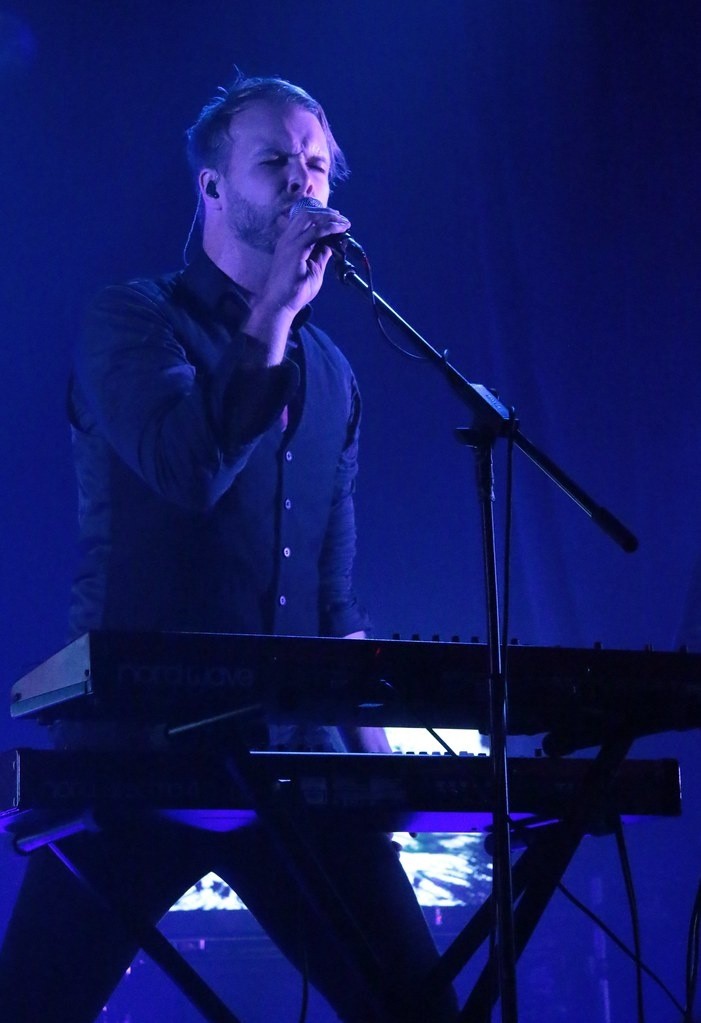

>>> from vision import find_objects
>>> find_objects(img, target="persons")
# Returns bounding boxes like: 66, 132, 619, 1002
0, 58, 457, 1023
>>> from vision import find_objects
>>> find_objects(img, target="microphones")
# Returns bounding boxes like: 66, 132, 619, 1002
289, 197, 367, 257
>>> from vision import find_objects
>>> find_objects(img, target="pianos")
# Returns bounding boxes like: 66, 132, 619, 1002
2, 631, 698, 1023
